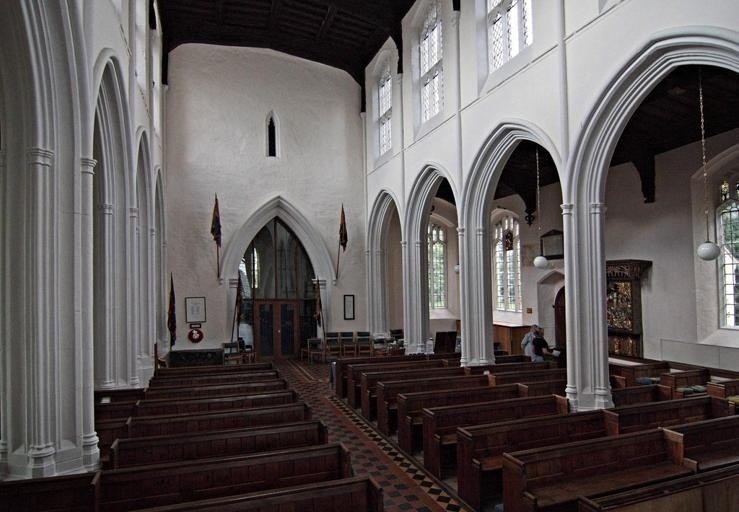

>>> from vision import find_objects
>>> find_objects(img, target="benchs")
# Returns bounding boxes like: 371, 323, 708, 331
1, 441, 385, 511
334, 354, 739, 512
97, 364, 330, 466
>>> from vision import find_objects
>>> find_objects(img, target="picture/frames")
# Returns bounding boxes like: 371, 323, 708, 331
186, 296, 206, 323
344, 294, 355, 320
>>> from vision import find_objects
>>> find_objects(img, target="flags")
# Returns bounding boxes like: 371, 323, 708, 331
339, 208, 349, 252
315, 281, 321, 327
209, 198, 224, 249
167, 279, 176, 350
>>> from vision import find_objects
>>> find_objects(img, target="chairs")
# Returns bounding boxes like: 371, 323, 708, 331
221, 337, 256, 366
298, 329, 404, 364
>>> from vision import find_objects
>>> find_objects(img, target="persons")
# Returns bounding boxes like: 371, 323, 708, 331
521, 324, 554, 362
